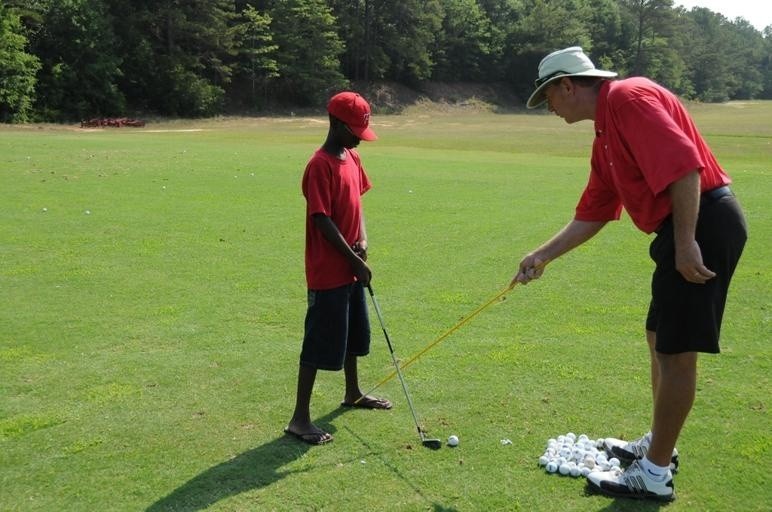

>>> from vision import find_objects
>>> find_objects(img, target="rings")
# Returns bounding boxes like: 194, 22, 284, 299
693, 271, 700, 277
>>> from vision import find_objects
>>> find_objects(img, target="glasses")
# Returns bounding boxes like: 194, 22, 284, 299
535, 70, 571, 88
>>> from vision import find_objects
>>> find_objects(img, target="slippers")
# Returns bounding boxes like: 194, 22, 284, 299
341, 397, 392, 409
284, 425, 333, 444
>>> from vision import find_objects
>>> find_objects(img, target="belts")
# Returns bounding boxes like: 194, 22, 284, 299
702, 186, 731, 207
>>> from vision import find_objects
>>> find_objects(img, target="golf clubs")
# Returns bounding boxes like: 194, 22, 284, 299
353, 243, 441, 448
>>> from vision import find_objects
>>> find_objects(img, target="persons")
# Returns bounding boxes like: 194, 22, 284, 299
509, 46, 748, 501
283, 91, 393, 446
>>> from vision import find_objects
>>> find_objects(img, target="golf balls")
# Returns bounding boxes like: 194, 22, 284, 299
448, 435, 458, 446
539, 433, 624, 477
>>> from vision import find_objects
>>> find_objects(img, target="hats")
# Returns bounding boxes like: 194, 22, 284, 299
327, 92, 377, 141
526, 46, 619, 109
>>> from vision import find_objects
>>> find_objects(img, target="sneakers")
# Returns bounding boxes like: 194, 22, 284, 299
586, 460, 674, 500
603, 431, 680, 474
